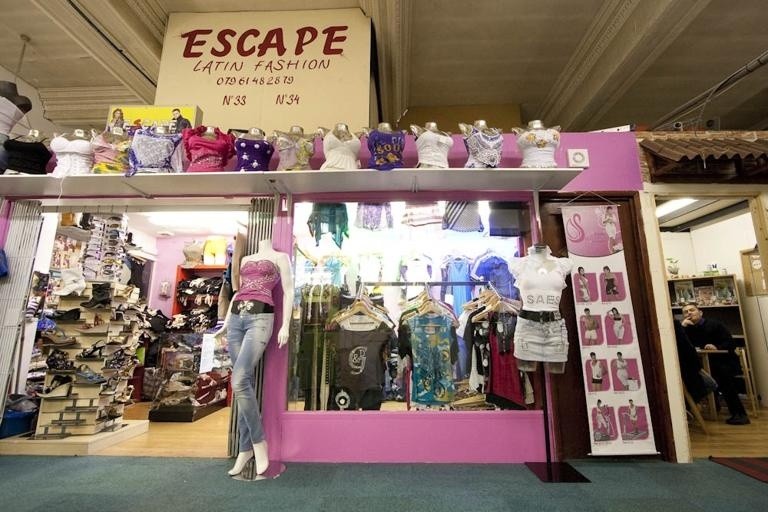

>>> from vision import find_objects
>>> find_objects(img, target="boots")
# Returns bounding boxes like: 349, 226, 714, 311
80, 284, 111, 309
52, 268, 86, 297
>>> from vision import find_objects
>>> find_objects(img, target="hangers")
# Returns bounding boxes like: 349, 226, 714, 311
404, 247, 506, 267
330, 281, 520, 328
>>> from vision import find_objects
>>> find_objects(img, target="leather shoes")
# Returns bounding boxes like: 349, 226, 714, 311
731, 416, 750, 425
725, 416, 733, 424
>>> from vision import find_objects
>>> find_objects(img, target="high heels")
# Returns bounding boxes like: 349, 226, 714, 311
99, 283, 152, 417
75, 364, 106, 384
41, 329, 76, 345
46, 349, 73, 370
75, 340, 106, 361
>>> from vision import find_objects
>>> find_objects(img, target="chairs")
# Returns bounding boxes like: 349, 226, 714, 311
730, 345, 757, 419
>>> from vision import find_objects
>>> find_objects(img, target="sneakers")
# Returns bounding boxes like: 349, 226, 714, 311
686, 410, 695, 421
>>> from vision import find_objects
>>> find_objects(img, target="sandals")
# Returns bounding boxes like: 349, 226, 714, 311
46, 308, 80, 320
34, 375, 72, 398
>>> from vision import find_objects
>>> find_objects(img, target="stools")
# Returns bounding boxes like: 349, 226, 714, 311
680, 379, 711, 439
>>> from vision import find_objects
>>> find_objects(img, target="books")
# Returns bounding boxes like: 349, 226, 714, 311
670, 277, 739, 306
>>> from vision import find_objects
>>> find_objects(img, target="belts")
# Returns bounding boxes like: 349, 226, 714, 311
517, 308, 561, 322
232, 301, 274, 315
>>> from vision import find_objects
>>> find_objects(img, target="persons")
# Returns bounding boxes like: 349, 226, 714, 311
578, 267, 590, 302
603, 265, 619, 302
602, 206, 617, 255
614, 351, 629, 391
596, 399, 608, 437
590, 351, 605, 392
511, 120, 561, 168
3, 129, 54, 174
318, 124, 364, 169
0, 80, 32, 174
410, 122, 453, 169
212, 238, 294, 477
362, 123, 407, 168
672, 311, 719, 427
681, 302, 752, 425
50, 121, 183, 175
171, 109, 192, 134
506, 242, 573, 376
111, 108, 125, 133
581, 308, 598, 345
184, 126, 314, 172
627, 399, 639, 435
459, 120, 504, 169
607, 307, 626, 345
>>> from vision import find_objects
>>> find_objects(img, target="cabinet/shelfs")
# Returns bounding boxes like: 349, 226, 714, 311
171, 261, 228, 315
667, 271, 758, 419
1, 227, 150, 454
146, 349, 229, 423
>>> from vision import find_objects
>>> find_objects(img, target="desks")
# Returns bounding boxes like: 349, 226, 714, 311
694, 348, 728, 420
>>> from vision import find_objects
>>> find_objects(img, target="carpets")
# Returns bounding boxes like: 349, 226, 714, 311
706, 453, 766, 484
